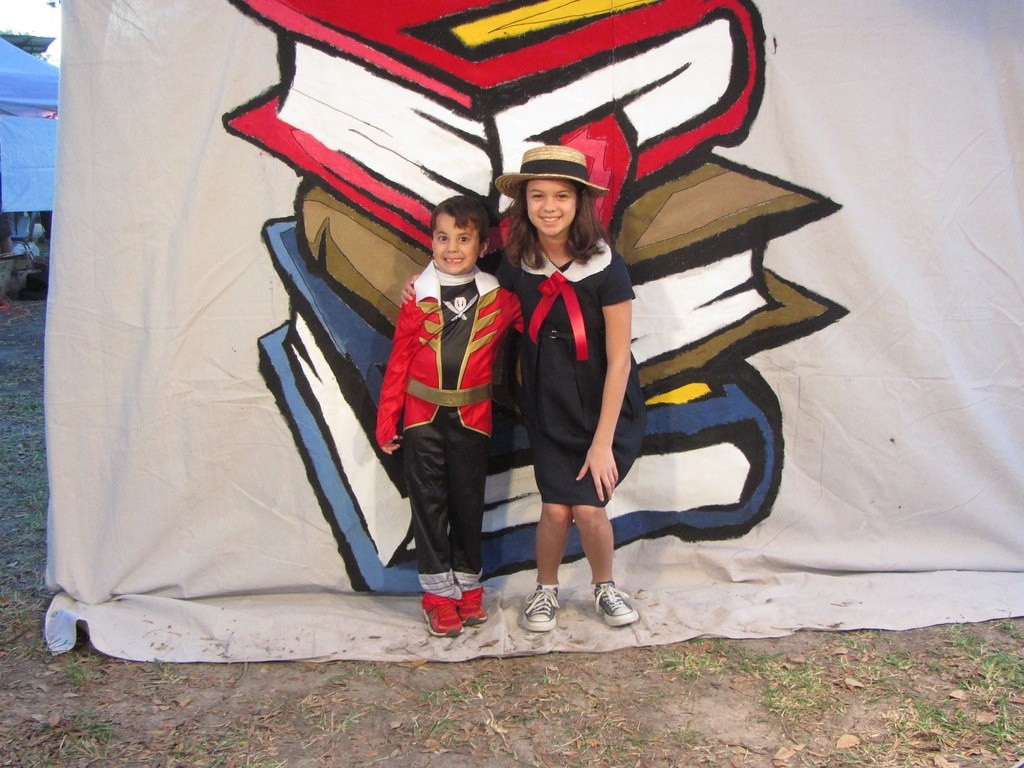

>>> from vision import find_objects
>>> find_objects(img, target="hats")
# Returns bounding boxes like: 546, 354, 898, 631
495, 143, 609, 201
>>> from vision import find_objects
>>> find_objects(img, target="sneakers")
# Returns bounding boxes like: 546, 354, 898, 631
522, 578, 560, 634
590, 580, 639, 628
457, 586, 487, 628
421, 589, 463, 639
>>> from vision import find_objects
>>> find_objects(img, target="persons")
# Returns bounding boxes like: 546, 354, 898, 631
398, 146, 647, 631
0, 197, 53, 258
376, 196, 523, 638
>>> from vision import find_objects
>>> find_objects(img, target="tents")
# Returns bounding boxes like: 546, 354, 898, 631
0, 37, 60, 213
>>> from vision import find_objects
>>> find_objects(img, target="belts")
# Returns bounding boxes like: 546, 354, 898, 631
540, 328, 576, 342
